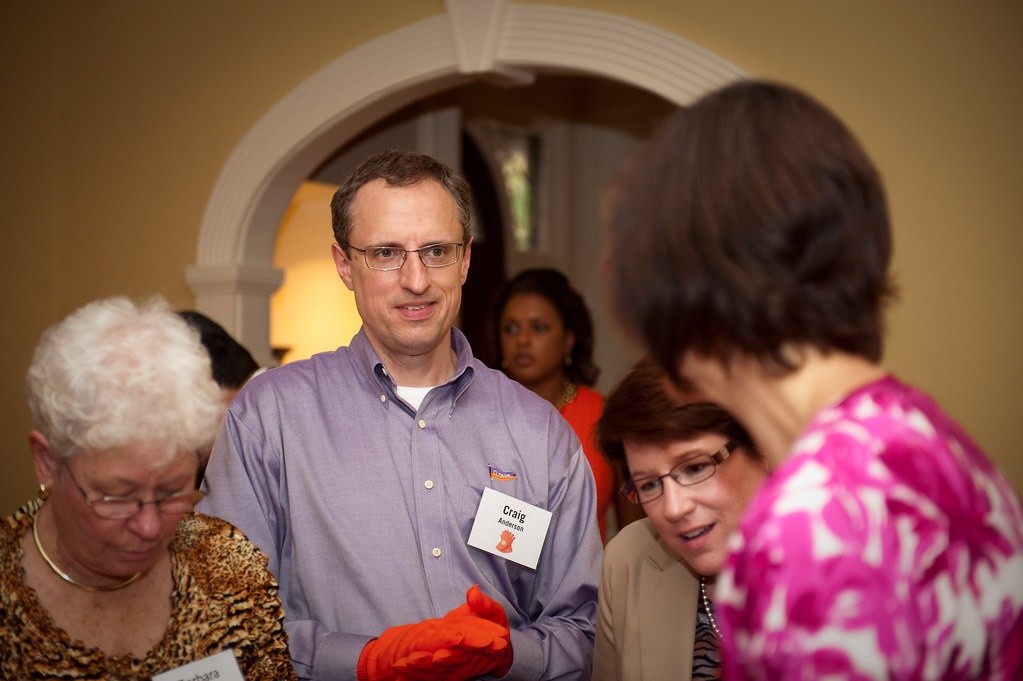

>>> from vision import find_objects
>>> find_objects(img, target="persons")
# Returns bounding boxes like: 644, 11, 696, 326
166, 307, 259, 492
588, 351, 776, 680
604, 77, 1022, 681
193, 145, 602, 681
1, 290, 294, 681
474, 265, 621, 548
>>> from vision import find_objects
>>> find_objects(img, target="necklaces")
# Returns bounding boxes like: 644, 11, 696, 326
29, 500, 149, 591
696, 572, 726, 643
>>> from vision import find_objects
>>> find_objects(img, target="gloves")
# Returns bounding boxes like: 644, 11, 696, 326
393, 584, 514, 681
357, 616, 508, 680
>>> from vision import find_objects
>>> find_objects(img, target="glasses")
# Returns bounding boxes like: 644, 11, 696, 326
620, 438, 741, 505
347, 240, 465, 270
61, 450, 211, 519
